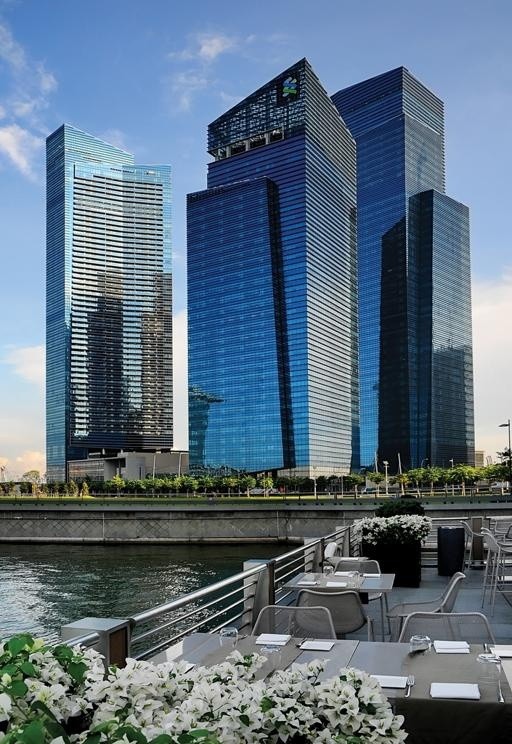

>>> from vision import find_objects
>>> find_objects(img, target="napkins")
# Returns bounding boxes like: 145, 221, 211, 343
428, 681, 483, 701
256, 633, 293, 644
433, 639, 473, 656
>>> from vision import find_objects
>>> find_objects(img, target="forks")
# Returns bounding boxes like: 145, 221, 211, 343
483, 643, 491, 655
405, 675, 414, 696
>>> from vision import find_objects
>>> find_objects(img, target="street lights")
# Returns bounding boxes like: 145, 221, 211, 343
383, 460, 389, 495
152, 451, 162, 481
498, 419, 512, 472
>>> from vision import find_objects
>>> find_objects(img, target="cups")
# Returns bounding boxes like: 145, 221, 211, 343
346, 570, 361, 588
219, 625, 238, 651
261, 644, 283, 675
410, 634, 432, 659
475, 652, 502, 681
323, 565, 334, 580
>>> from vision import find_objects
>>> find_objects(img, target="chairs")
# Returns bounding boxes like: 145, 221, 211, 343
251, 559, 497, 644
461, 514, 512, 617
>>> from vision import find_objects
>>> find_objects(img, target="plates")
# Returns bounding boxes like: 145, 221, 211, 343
369, 674, 409, 690
334, 571, 351, 577
326, 582, 347, 588
299, 639, 336, 651
490, 647, 512, 657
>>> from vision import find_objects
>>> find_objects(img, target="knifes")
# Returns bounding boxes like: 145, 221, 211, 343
497, 679, 505, 704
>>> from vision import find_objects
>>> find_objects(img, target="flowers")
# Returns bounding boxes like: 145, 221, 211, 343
354, 513, 434, 548
0, 631, 411, 744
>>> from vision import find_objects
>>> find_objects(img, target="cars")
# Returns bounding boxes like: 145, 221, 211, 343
246, 488, 278, 494
441, 485, 461, 490
489, 481, 508, 489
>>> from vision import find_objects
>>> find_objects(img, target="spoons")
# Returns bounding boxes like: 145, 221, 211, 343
296, 637, 309, 647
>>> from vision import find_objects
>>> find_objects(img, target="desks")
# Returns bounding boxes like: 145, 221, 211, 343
144, 631, 359, 693
338, 640, 511, 743
360, 528, 422, 589
